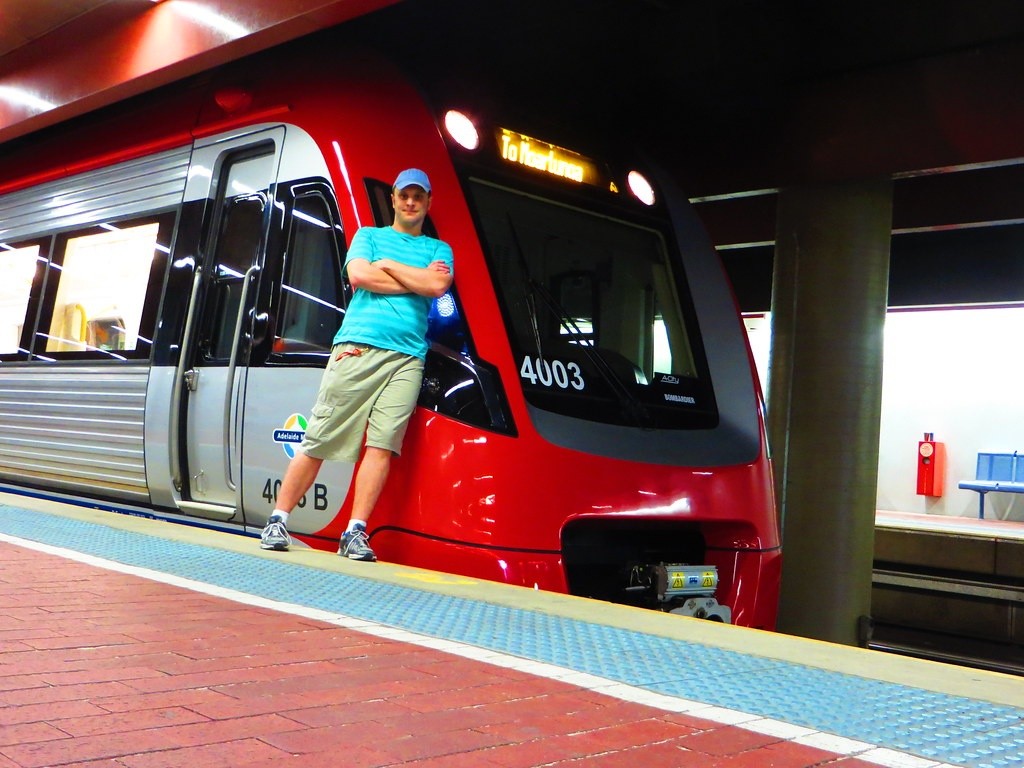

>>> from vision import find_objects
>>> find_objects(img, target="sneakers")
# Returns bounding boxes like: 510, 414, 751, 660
259, 515, 293, 551
336, 523, 378, 562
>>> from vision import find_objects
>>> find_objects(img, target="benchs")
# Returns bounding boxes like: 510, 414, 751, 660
958, 452, 1024, 520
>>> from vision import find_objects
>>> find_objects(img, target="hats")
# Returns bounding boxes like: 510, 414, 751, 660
392, 167, 432, 193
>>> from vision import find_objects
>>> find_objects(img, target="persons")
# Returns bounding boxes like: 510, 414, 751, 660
259, 168, 455, 562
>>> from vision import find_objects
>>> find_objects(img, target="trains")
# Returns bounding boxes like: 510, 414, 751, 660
0, 49, 784, 640
